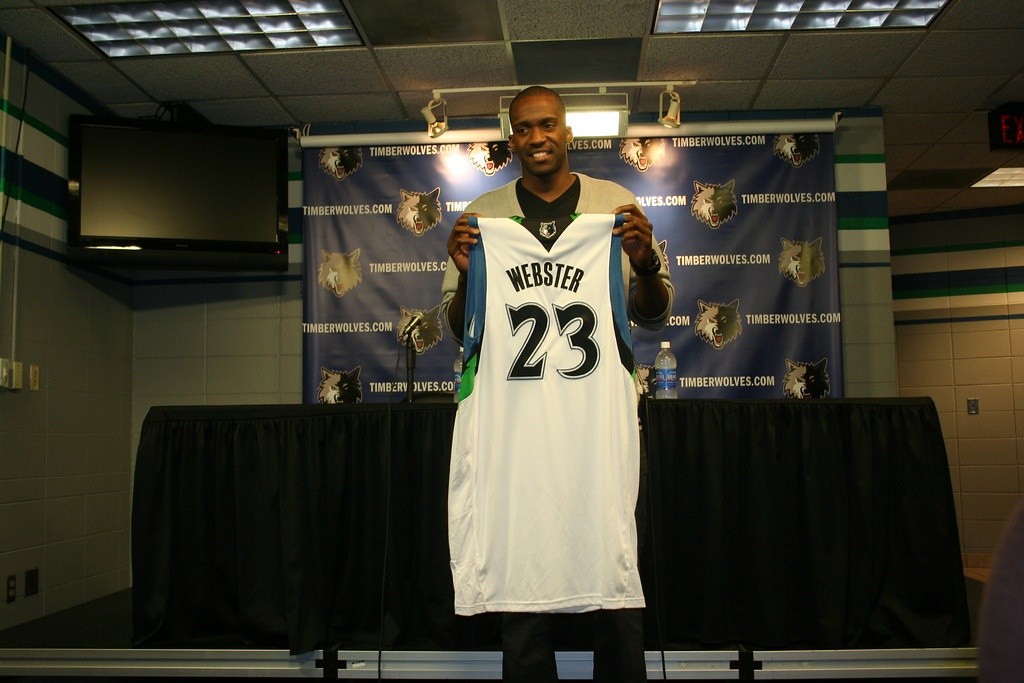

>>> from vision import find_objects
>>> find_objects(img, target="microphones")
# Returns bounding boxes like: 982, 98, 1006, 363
403, 311, 424, 335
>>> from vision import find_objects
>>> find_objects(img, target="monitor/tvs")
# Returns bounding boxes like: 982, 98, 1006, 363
71, 116, 289, 255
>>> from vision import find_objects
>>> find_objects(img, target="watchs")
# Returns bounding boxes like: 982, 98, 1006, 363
629, 249, 661, 277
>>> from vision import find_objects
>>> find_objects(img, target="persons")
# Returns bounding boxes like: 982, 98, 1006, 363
440, 86, 674, 679
